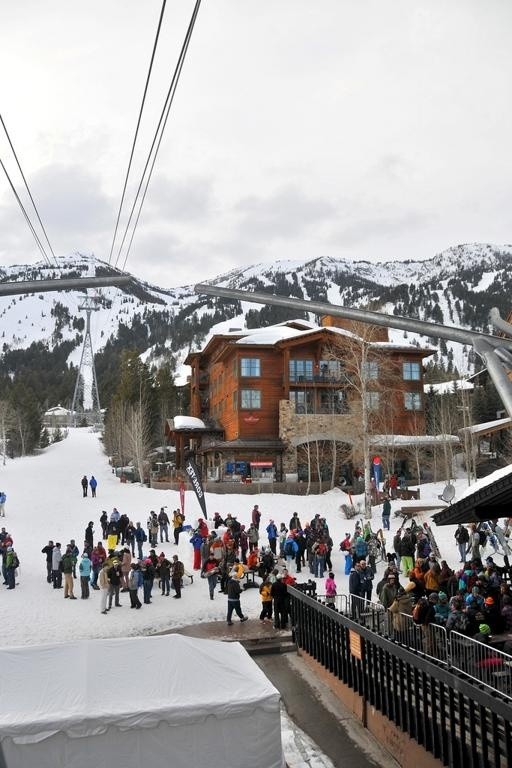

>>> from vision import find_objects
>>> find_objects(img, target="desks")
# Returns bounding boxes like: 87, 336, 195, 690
241, 569, 259, 589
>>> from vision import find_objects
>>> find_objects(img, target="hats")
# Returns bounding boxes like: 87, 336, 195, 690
388, 572, 395, 583
484, 596, 493, 605
428, 552, 435, 558
438, 591, 446, 601
479, 624, 491, 634
472, 587, 479, 592
430, 592, 441, 601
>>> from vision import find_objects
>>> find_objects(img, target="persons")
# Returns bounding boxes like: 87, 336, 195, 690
0, 526, 20, 590
190, 504, 334, 629
43, 503, 185, 616
0, 491, 7, 517
89, 476, 98, 498
380, 474, 391, 495
382, 495, 391, 530
390, 473, 397, 500
82, 475, 88, 497
326, 519, 511, 682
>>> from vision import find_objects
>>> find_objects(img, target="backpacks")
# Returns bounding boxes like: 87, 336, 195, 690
455, 614, 471, 633
413, 598, 427, 625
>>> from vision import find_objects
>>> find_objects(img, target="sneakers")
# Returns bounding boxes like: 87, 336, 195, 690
227, 622, 233, 625
240, 617, 248, 623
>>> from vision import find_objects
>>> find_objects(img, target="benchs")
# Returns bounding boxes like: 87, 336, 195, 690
155, 566, 197, 588
359, 604, 512, 693
